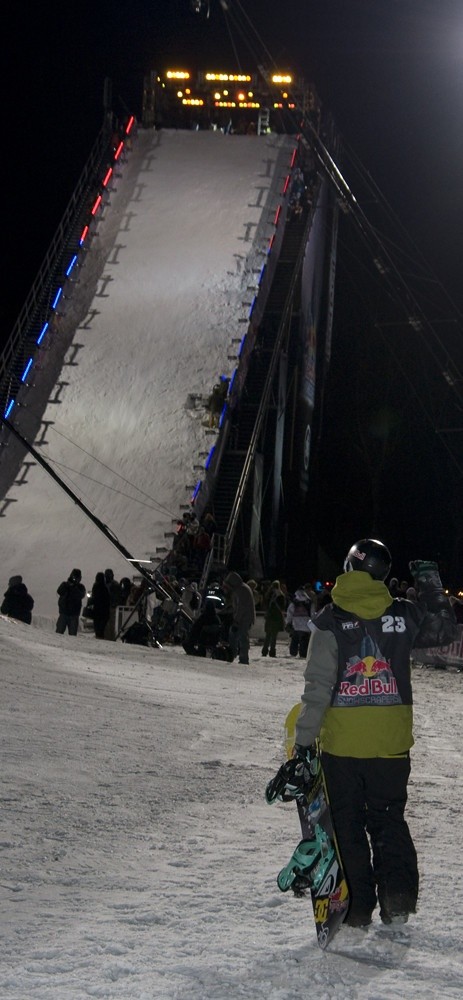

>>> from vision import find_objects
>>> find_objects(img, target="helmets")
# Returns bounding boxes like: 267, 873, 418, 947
344, 539, 393, 581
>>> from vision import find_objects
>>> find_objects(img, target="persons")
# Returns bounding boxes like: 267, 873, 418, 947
202, 513, 217, 539
0, 576, 34, 625
283, 150, 321, 230
228, 407, 239, 451
293, 538, 458, 953
87, 568, 463, 665
191, 527, 213, 568
56, 569, 86, 637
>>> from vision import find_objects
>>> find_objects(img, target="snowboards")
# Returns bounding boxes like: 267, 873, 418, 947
281, 703, 350, 950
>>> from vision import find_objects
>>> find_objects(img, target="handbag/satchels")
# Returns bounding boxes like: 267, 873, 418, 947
121, 621, 151, 647
208, 641, 236, 664
83, 605, 94, 619
292, 616, 311, 633
190, 592, 200, 611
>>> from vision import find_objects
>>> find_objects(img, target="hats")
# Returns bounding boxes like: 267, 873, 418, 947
295, 591, 309, 602
407, 588, 416, 595
105, 569, 114, 577
247, 580, 257, 589
9, 576, 22, 585
305, 583, 313, 589
71, 569, 81, 578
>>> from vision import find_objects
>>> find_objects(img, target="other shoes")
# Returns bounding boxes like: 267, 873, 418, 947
289, 656, 296, 658
344, 913, 372, 928
296, 657, 306, 660
380, 907, 407, 924
